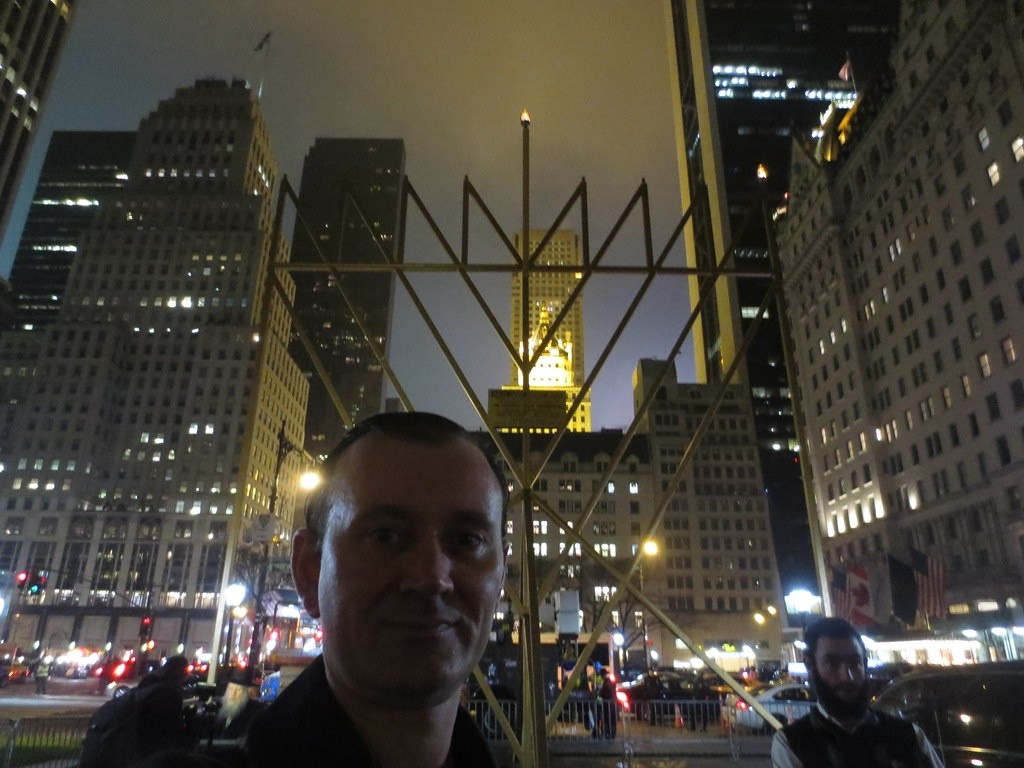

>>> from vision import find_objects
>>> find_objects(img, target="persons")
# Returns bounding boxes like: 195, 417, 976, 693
28, 650, 52, 694
771, 617, 945, 768
742, 664, 788, 682
642, 667, 664, 724
588, 662, 620, 738
81, 411, 510, 768
689, 670, 710, 732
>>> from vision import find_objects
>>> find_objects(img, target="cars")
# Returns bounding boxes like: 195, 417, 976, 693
105, 671, 211, 712
616, 660, 1024, 768
0, 642, 31, 688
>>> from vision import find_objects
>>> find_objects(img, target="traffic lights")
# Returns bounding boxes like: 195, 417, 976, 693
29, 577, 47, 596
139, 614, 150, 638
17, 574, 27, 583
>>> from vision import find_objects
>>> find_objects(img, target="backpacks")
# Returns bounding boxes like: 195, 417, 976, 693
79, 683, 163, 768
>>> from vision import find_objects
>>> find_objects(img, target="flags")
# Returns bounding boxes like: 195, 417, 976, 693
832, 567, 874, 625
887, 549, 943, 626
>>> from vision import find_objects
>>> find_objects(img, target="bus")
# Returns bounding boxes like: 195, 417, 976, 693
460, 627, 613, 740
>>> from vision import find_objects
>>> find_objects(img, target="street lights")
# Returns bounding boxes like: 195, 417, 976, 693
247, 419, 321, 683
221, 584, 246, 667
639, 540, 659, 671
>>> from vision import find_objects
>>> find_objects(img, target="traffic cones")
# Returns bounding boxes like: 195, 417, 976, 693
674, 703, 685, 729
